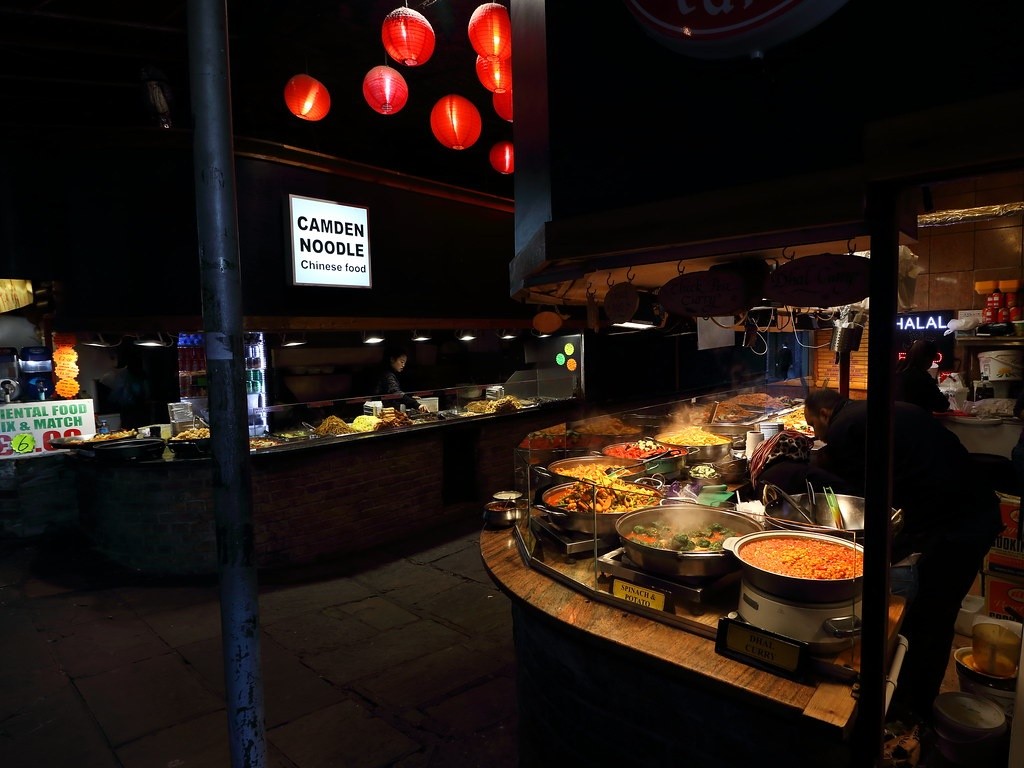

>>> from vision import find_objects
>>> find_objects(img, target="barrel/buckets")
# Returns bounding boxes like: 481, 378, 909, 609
931, 691, 1008, 764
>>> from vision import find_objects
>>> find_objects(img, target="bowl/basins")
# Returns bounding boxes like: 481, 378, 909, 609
46, 430, 210, 461
483, 500, 517, 529
954, 647, 1019, 692
492, 490, 523, 503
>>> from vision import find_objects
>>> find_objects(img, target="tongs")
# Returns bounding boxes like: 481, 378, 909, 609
264, 430, 289, 442
192, 414, 210, 428
302, 422, 316, 432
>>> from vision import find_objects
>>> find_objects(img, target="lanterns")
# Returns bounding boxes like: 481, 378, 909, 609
280, 2, 515, 175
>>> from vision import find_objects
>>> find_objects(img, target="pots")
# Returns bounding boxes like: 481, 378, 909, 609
978, 350, 1024, 382
533, 404, 902, 655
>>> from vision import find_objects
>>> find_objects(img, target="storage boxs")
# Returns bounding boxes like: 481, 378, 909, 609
984, 491, 1024, 623
954, 594, 986, 636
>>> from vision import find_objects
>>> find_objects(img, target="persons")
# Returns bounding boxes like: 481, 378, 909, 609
803, 388, 1003, 725
895, 337, 951, 414
371, 343, 426, 413
1010, 396, 1024, 543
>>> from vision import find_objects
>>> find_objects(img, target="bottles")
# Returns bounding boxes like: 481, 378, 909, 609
98, 419, 110, 434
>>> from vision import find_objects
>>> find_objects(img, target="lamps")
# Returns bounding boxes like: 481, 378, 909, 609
455, 328, 478, 341
362, 330, 384, 343
412, 329, 431, 341
281, 332, 308, 347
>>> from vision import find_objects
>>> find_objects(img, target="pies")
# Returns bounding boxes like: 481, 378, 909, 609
379, 407, 395, 419
